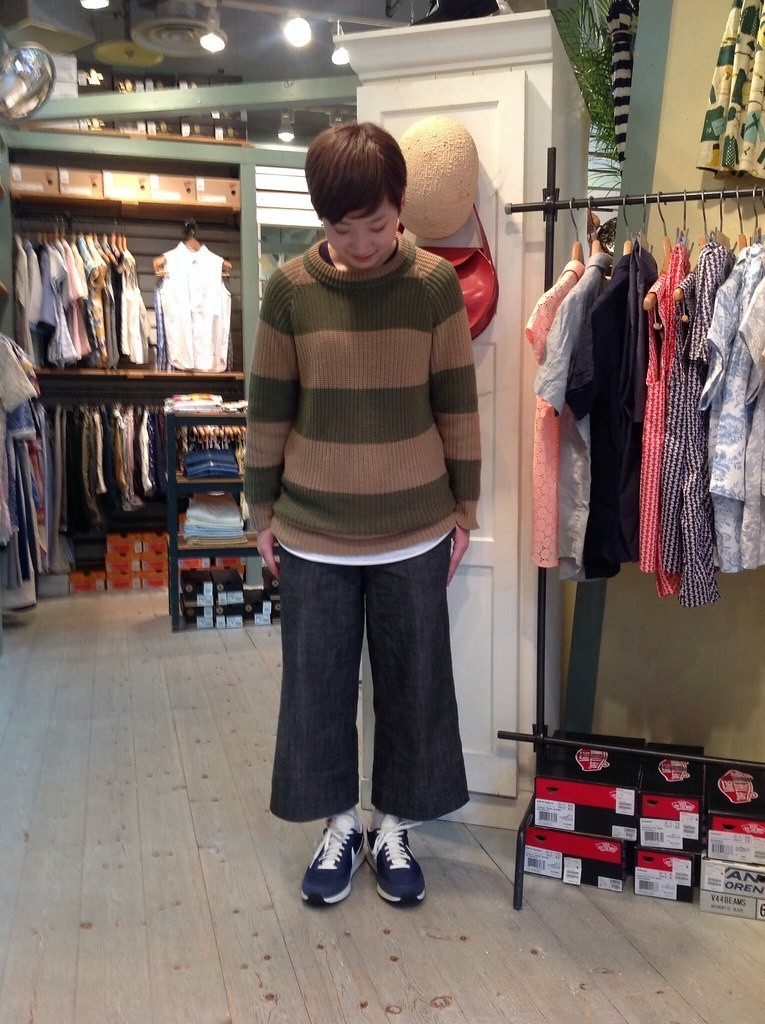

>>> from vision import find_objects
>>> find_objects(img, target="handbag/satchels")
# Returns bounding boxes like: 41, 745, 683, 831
399, 197, 499, 340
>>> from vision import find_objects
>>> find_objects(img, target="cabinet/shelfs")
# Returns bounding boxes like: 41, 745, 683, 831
11, 197, 245, 384
165, 411, 285, 632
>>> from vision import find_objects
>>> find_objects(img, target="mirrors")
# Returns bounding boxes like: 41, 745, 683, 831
251, 163, 325, 310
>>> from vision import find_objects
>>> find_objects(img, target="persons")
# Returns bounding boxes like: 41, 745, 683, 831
243, 123, 483, 906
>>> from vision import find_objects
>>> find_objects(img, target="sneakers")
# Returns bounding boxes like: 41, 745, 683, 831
367, 814, 425, 905
301, 812, 366, 905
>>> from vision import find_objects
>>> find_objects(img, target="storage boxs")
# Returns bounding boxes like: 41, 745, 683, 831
521, 728, 765, 922
68, 512, 283, 628
35, 52, 249, 144
8, 164, 241, 207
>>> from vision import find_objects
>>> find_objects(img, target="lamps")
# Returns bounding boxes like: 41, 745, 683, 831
199, 0, 227, 53
277, 109, 296, 142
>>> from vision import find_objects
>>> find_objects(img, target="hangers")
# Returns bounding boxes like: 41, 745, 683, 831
568, 186, 765, 307
49, 395, 247, 440
151, 218, 233, 279
12, 218, 135, 266
